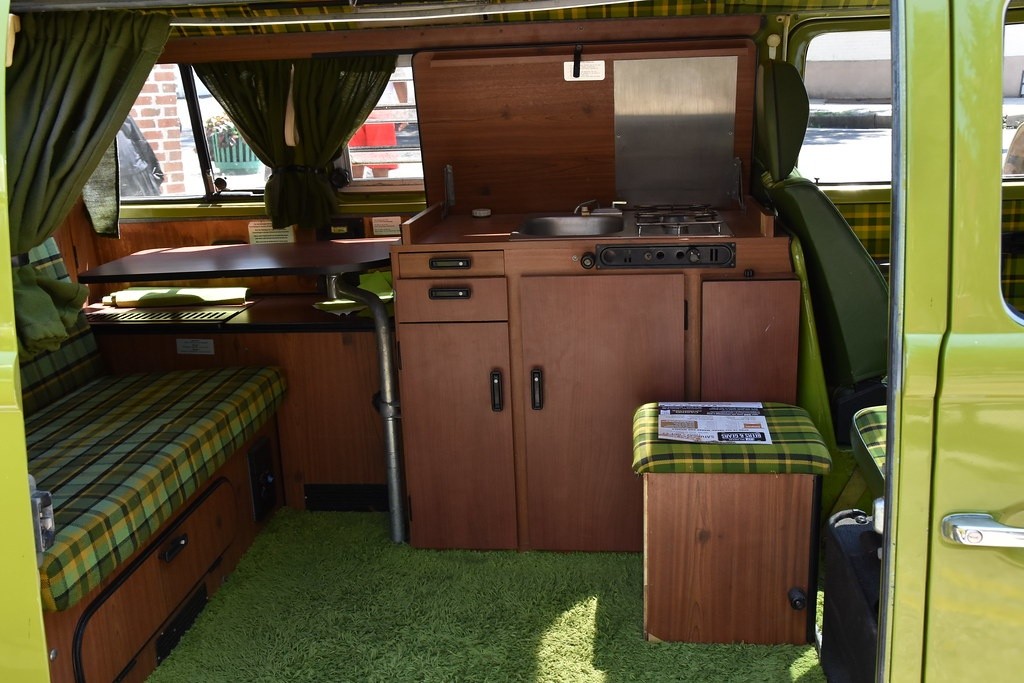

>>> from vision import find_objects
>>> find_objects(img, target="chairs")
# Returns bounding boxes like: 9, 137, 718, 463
756, 58, 889, 394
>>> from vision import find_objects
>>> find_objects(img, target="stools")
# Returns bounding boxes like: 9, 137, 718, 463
633, 400, 833, 644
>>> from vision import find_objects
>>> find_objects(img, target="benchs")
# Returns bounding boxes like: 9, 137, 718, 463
11, 236, 288, 683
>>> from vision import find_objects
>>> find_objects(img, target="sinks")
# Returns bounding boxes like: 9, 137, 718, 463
507, 213, 627, 244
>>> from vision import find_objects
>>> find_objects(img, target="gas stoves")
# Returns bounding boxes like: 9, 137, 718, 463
634, 206, 735, 238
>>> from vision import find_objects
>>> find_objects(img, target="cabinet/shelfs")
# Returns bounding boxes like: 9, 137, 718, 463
77, 236, 403, 506
389, 203, 802, 554
